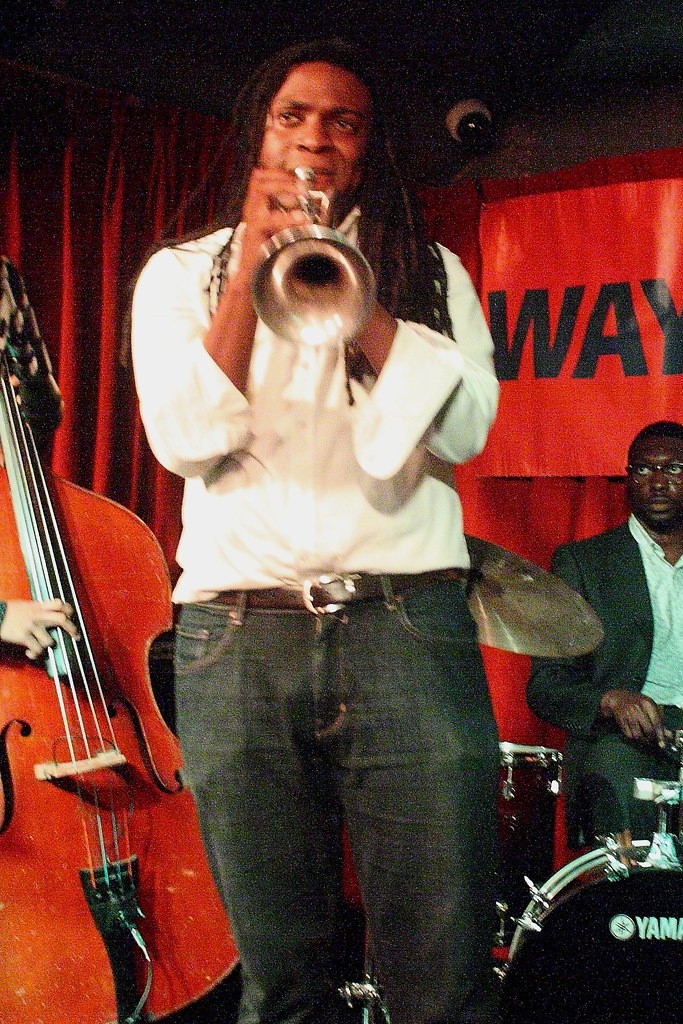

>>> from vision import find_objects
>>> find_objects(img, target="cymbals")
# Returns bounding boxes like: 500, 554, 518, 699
463, 533, 603, 659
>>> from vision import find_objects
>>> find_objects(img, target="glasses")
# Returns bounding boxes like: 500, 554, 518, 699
627, 460, 683, 486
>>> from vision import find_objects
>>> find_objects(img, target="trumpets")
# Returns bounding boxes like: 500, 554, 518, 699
251, 166, 374, 349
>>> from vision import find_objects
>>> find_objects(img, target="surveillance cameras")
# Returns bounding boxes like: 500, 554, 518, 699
445, 99, 494, 147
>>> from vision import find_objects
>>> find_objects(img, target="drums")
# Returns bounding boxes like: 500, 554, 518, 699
495, 743, 564, 946
494, 836, 683, 1023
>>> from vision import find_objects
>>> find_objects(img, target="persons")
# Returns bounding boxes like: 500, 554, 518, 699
127, 33, 503, 1024
528, 421, 683, 856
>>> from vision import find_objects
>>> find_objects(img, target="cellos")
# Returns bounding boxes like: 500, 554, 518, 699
0, 253, 241, 1023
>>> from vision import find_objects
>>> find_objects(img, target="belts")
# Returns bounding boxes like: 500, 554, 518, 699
212, 572, 461, 610
